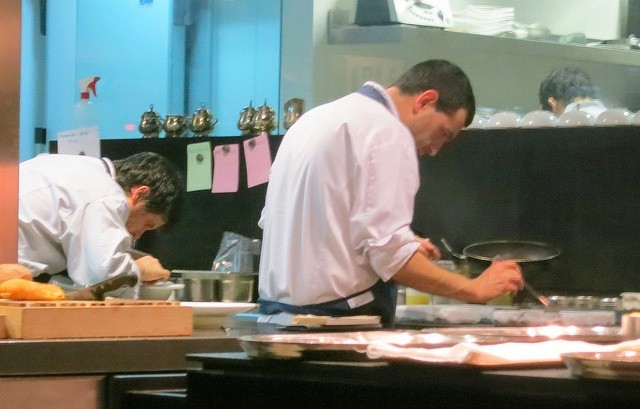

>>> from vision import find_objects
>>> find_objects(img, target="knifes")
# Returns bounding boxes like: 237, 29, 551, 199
67, 270, 139, 300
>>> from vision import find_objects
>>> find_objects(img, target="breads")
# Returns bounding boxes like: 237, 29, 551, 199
0, 278, 65, 300
0, 261, 32, 280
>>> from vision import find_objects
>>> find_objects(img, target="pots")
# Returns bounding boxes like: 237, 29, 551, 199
454, 241, 560, 277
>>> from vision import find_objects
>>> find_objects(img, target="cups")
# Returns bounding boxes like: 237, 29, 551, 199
601, 296, 622, 324
284, 98, 304, 132
574, 295, 598, 310
547, 294, 572, 312
251, 99, 275, 135
238, 100, 255, 136
138, 103, 164, 138
162, 115, 191, 137
188, 101, 218, 136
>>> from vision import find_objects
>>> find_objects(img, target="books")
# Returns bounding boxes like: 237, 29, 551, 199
325, 314, 382, 326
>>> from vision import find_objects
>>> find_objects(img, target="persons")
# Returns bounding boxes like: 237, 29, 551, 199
18, 150, 186, 298
258, 60, 525, 329
539, 65, 608, 120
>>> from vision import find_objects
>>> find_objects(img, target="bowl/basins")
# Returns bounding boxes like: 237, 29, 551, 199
595, 107, 634, 126
174, 279, 219, 300
631, 110, 640, 124
218, 278, 256, 302
140, 281, 173, 300
522, 110, 558, 128
485, 111, 521, 128
463, 113, 484, 128
557, 111, 595, 127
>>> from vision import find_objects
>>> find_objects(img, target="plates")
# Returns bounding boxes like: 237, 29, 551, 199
182, 302, 255, 330
